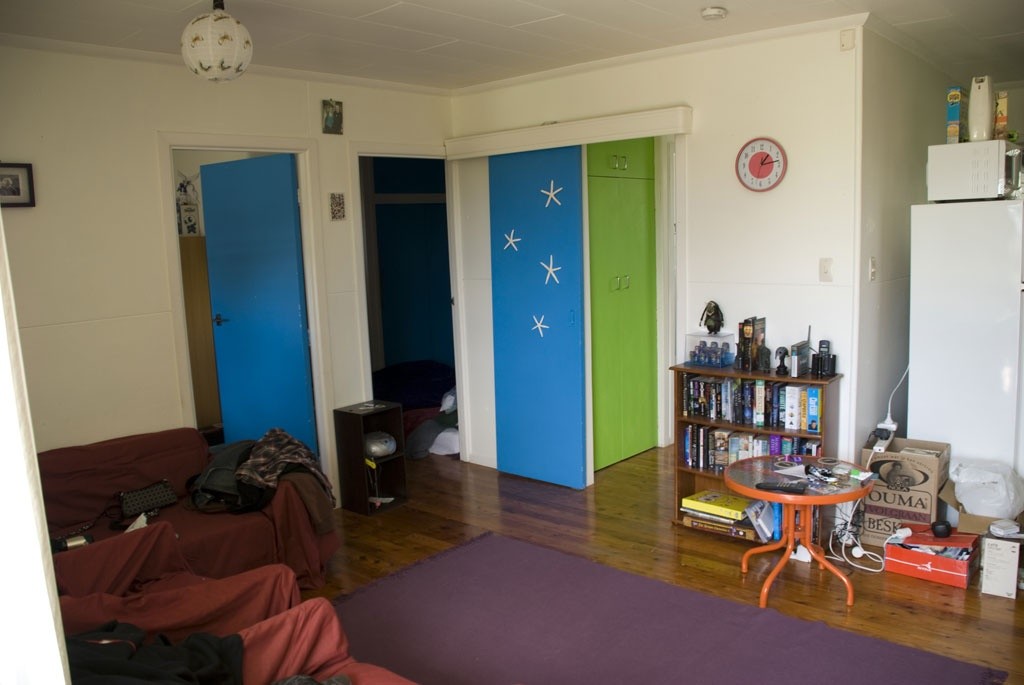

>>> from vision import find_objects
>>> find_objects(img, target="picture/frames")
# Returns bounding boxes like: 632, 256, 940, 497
0, 163, 35, 207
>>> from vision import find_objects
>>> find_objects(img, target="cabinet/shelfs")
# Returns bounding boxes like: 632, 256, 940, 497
671, 361, 844, 544
333, 400, 411, 515
483, 139, 659, 490
178, 232, 221, 426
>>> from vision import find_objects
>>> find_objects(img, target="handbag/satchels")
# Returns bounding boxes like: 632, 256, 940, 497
948, 458, 1024, 519
186, 439, 279, 515
122, 479, 178, 518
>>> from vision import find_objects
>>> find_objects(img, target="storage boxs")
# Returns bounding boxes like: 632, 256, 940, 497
861, 436, 1024, 599
684, 331, 735, 368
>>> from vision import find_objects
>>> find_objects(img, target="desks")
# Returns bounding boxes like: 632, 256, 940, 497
725, 455, 876, 609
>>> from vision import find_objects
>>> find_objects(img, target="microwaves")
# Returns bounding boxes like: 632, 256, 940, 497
926, 139, 1024, 204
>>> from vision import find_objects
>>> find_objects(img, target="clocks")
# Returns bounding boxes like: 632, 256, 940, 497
736, 138, 788, 191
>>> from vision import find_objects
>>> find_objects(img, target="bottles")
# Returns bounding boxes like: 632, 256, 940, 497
55, 534, 93, 552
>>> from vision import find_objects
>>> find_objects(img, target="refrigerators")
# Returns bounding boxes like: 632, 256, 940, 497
905, 202, 1023, 526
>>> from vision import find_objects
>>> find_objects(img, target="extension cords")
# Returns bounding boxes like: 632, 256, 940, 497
873, 430, 895, 453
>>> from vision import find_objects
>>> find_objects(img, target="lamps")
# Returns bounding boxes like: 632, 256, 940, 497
181, 0, 254, 81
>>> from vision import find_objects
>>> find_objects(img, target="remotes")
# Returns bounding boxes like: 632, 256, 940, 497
755, 482, 805, 493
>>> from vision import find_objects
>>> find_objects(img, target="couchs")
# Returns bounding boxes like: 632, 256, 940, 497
36, 425, 418, 685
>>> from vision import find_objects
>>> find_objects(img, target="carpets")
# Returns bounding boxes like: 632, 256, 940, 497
331, 531, 1007, 685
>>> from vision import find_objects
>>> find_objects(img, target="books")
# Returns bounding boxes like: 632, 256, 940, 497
680, 373, 826, 471
680, 489, 781, 543
791, 341, 809, 378
742, 316, 766, 370
